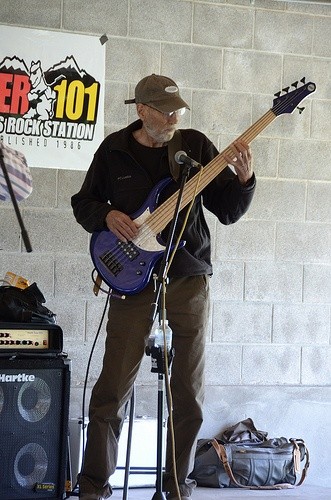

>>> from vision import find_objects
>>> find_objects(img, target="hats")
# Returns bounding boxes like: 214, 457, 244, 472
125, 73, 190, 113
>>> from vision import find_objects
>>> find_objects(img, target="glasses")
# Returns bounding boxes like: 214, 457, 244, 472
145, 104, 187, 119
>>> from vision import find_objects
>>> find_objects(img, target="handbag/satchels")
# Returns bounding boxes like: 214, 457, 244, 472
188, 420, 311, 491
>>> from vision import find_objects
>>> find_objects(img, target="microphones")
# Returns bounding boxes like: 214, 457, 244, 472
175, 150, 202, 168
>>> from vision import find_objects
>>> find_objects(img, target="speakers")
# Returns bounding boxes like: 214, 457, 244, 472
0, 355, 71, 500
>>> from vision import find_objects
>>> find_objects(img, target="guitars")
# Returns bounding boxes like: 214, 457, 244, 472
89, 75, 316, 295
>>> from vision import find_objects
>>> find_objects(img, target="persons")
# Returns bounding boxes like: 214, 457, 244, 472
71, 73, 256, 500
